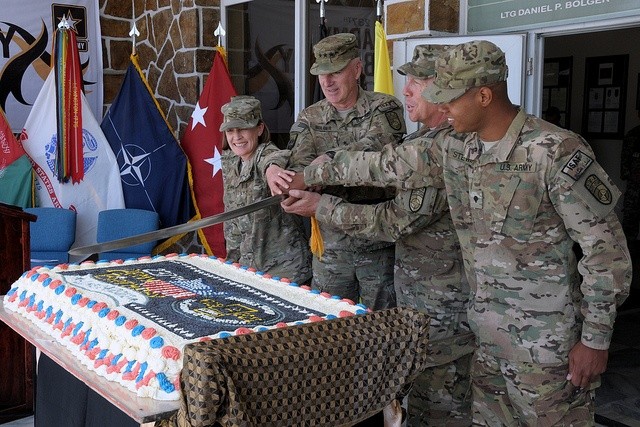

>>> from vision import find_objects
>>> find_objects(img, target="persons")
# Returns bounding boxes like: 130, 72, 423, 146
283, 32, 407, 315
221, 95, 312, 291
279, 37, 634, 426
279, 44, 473, 427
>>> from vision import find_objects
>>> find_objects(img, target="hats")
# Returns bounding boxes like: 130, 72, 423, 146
397, 45, 444, 80
422, 39, 509, 105
310, 32, 362, 76
219, 97, 264, 133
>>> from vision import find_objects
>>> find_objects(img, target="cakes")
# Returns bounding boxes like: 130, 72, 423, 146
1, 251, 373, 402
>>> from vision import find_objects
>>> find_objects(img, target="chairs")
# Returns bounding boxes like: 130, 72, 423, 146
96, 208, 159, 260
21, 207, 77, 266
155, 305, 430, 427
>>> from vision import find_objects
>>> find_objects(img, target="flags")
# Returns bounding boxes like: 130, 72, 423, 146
17, 26, 124, 255
98, 50, 199, 267
182, 45, 240, 255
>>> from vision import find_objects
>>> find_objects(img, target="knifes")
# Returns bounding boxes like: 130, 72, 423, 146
68, 191, 288, 257
